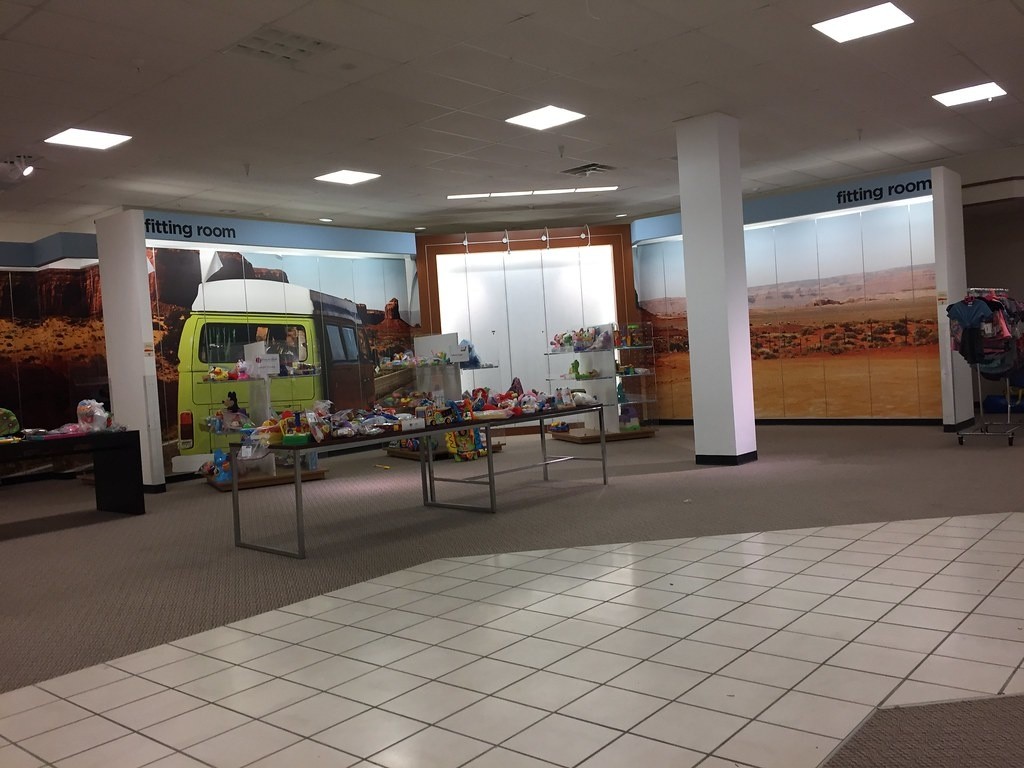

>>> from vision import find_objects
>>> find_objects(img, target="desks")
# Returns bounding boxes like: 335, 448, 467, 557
228, 419, 496, 559
0, 428, 145, 516
428, 402, 608, 512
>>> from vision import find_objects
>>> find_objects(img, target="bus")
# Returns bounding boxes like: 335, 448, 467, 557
169, 278, 380, 474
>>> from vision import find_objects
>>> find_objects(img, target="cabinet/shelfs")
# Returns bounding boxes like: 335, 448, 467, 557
544, 320, 661, 445
379, 348, 458, 459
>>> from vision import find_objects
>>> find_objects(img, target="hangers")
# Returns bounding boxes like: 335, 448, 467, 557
958, 288, 1011, 303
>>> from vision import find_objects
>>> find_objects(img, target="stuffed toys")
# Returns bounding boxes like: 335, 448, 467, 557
549, 328, 611, 380
517, 393, 549, 411
221, 391, 240, 413
208, 359, 250, 381
